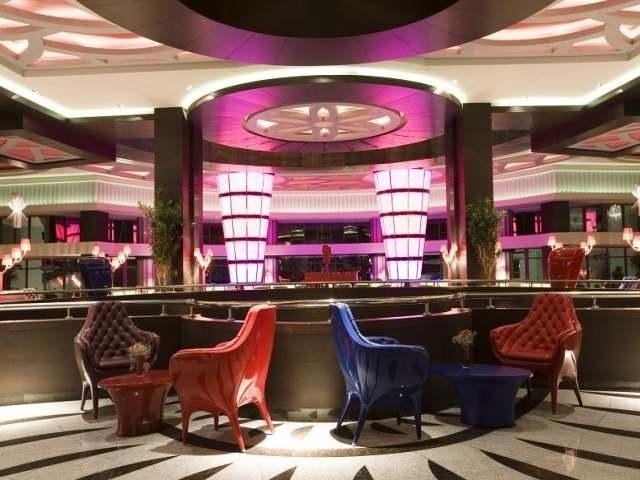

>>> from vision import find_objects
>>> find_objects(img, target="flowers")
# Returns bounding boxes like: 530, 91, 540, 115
452, 330, 477, 348
129, 343, 150, 360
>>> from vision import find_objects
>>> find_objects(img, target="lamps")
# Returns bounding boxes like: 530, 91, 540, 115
193, 247, 213, 284
0, 238, 32, 291
90, 244, 132, 287
622, 228, 640, 252
439, 244, 458, 279
547, 234, 596, 256
217, 172, 273, 283
372, 169, 432, 280
6, 191, 29, 229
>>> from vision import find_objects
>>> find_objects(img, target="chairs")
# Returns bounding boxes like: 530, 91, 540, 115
490, 294, 583, 415
328, 302, 430, 447
169, 305, 277, 452
74, 301, 160, 419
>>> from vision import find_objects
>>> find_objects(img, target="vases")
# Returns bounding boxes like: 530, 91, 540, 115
464, 346, 470, 367
136, 357, 144, 378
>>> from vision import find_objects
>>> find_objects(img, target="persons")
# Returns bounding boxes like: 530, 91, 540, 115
612, 265, 626, 280
